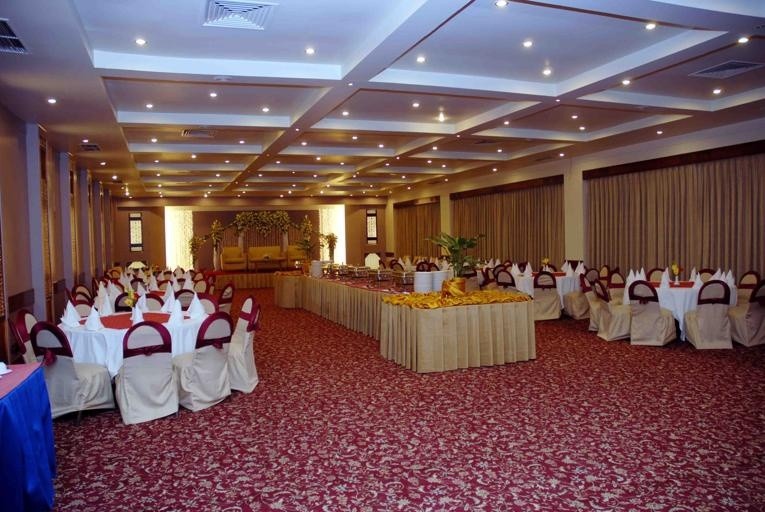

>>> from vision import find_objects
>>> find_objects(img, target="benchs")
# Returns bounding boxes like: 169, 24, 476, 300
247, 246, 286, 270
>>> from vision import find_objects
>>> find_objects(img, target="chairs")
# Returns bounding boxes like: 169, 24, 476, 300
281, 245, 308, 268
220, 246, 247, 269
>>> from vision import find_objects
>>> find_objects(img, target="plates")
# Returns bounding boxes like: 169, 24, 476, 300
432, 270, 450, 292
310, 260, 324, 277
413, 271, 433, 294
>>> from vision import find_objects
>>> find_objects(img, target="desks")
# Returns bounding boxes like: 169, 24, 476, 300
272, 263, 539, 372
0, 360, 57, 511
